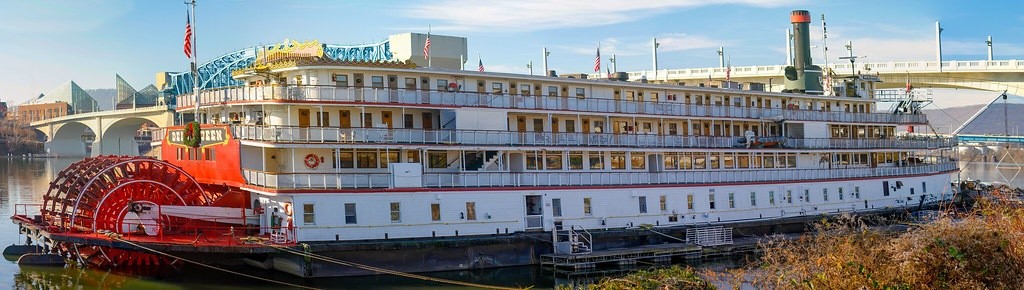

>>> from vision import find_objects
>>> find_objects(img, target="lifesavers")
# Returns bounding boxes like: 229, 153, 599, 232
285, 202, 292, 215
305, 153, 320, 169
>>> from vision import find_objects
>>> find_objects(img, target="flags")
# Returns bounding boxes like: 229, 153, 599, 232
826, 69, 830, 88
478, 59, 483, 72
595, 48, 600, 72
183, 9, 192, 58
607, 68, 612, 79
424, 31, 430, 59
726, 58, 730, 80
